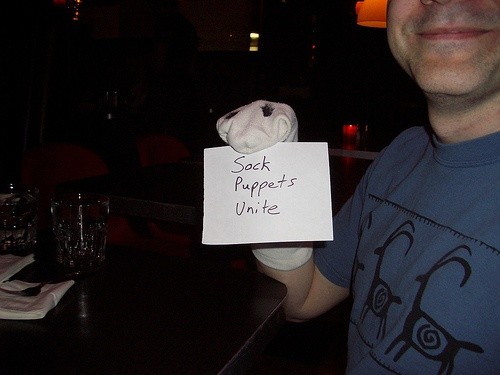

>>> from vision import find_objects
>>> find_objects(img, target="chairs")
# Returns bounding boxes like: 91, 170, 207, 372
22, 145, 185, 257
133, 132, 204, 245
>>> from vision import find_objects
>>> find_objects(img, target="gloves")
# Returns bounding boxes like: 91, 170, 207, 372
211, 98, 313, 273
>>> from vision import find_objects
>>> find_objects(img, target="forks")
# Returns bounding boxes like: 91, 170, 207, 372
0, 274, 62, 297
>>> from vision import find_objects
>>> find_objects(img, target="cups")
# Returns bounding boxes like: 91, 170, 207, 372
49, 194, 110, 274
0, 182, 41, 255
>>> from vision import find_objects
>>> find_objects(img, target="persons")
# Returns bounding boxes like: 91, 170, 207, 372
216, 0, 500, 375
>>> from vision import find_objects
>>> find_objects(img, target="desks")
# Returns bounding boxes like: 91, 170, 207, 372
0, 243, 289, 375
